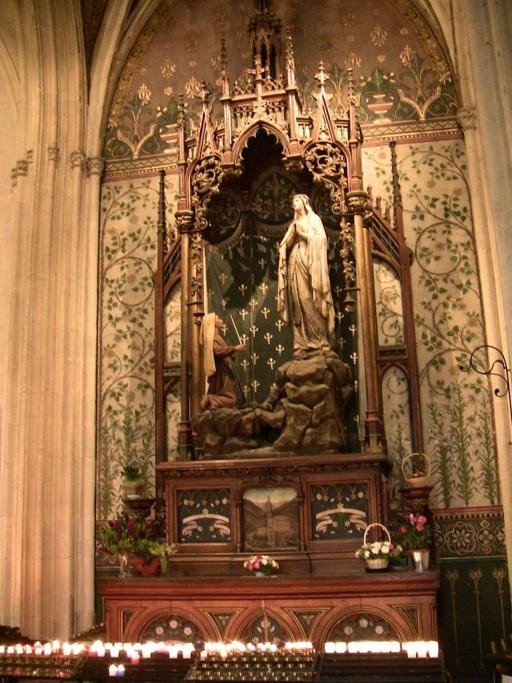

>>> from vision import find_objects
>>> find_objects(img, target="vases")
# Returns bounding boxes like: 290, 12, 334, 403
129, 551, 162, 575
408, 548, 430, 570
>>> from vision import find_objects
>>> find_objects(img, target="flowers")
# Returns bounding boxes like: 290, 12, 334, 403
353, 540, 403, 560
92, 512, 175, 558
242, 555, 279, 571
394, 512, 432, 549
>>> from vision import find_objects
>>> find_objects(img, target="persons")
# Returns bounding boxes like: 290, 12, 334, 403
274, 192, 338, 359
194, 308, 248, 408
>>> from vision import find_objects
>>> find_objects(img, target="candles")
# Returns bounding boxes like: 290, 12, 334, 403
108, 664, 126, 678
